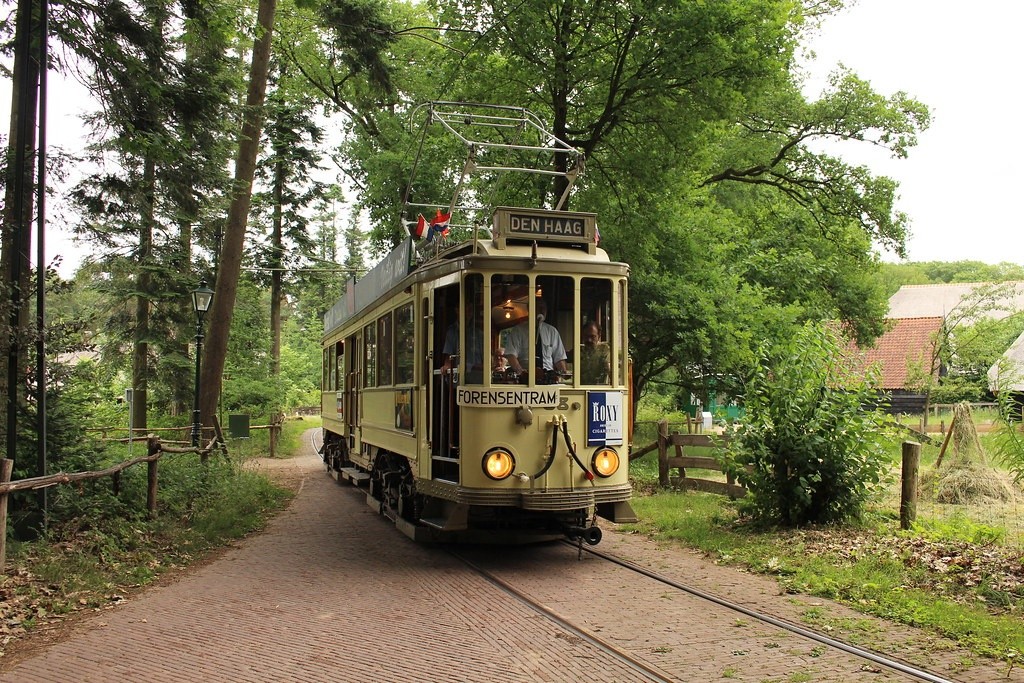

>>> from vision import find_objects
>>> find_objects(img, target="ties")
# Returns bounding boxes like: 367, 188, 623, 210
535, 333, 543, 370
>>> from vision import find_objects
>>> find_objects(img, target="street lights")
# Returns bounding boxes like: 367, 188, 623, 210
189, 277, 216, 448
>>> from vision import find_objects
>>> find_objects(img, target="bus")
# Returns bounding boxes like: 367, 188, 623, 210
318, 99, 643, 546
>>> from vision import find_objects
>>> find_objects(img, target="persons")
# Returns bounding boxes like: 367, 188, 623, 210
398, 333, 414, 367
580, 321, 611, 385
503, 299, 567, 384
491, 348, 512, 378
385, 350, 392, 367
441, 301, 484, 384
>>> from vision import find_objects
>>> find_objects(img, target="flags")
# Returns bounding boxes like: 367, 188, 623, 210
415, 215, 433, 242
430, 210, 450, 239
